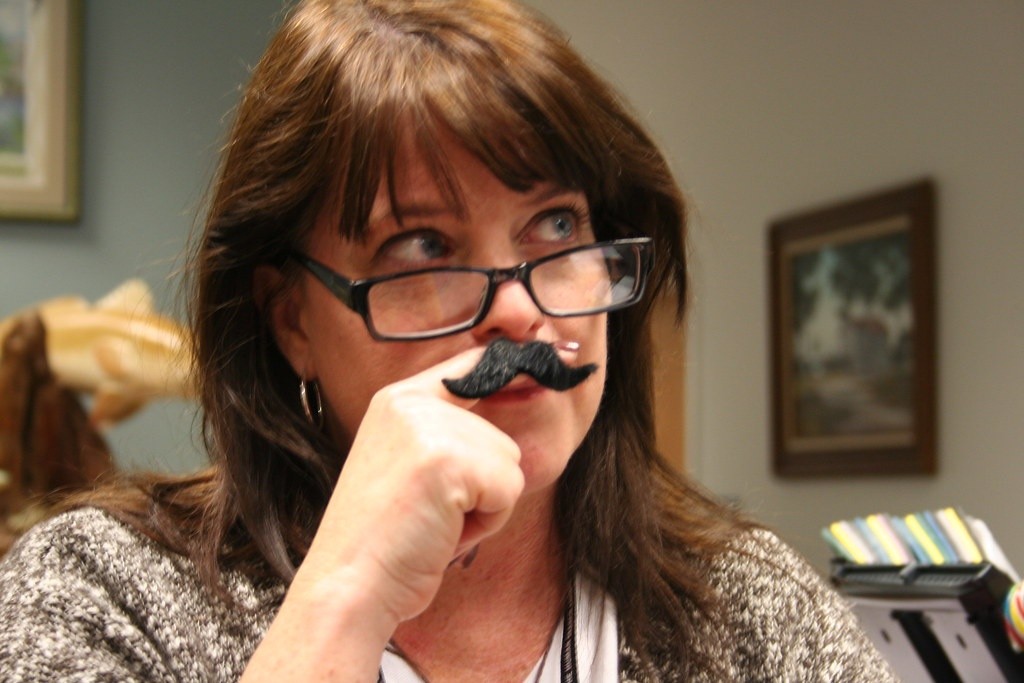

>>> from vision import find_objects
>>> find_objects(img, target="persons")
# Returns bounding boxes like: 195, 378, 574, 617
0, 0, 904, 683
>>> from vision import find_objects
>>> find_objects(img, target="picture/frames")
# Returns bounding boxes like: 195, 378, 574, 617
764, 180, 933, 478
0, 1, 83, 226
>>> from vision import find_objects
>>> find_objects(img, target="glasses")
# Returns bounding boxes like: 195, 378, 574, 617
294, 235, 652, 341
